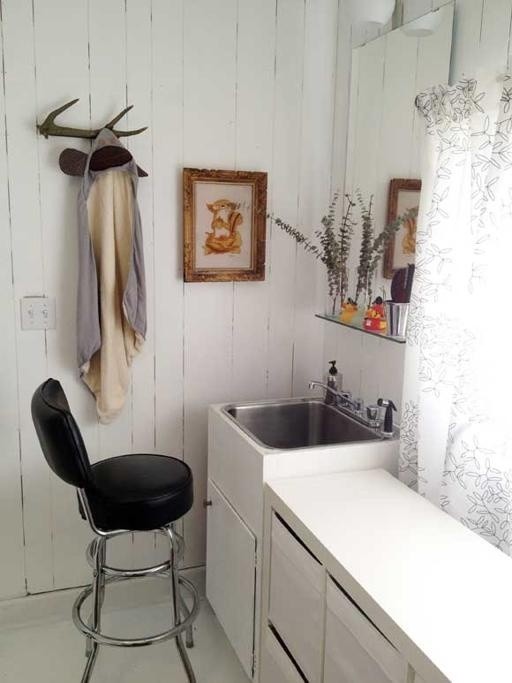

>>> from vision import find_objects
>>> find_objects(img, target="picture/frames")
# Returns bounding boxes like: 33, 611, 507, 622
182, 166, 268, 283
382, 177, 423, 279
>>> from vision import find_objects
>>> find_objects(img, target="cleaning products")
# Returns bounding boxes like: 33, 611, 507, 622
324, 360, 344, 406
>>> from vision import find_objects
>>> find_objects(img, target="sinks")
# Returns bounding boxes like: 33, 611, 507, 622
220, 396, 389, 454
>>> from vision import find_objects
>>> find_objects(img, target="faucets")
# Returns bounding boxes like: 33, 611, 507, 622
377, 398, 397, 433
308, 380, 363, 412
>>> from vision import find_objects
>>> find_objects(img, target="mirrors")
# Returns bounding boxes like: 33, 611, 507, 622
347, 1, 461, 325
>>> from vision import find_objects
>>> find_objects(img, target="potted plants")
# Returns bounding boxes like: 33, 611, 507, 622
384, 263, 416, 338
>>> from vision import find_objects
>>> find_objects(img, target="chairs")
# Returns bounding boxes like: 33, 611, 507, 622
29, 374, 210, 682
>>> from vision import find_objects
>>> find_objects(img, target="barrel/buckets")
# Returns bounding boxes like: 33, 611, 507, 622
380, 297, 410, 337
